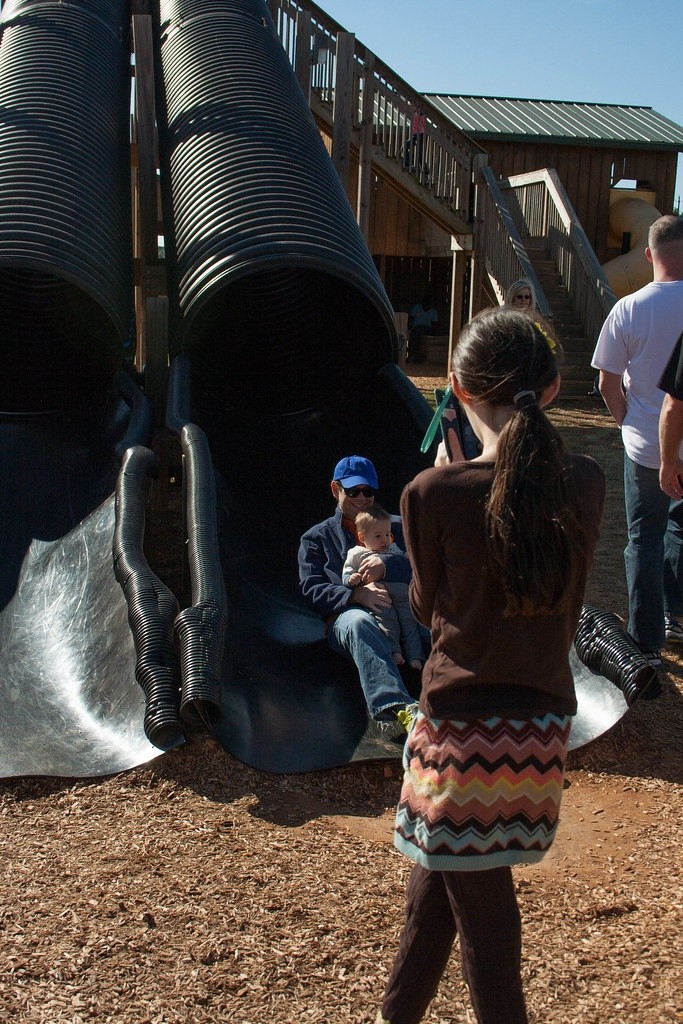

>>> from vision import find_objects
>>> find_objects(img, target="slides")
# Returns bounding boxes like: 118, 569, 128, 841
0, 0, 660, 783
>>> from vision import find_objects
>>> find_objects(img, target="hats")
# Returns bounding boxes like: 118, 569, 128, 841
333, 455, 378, 490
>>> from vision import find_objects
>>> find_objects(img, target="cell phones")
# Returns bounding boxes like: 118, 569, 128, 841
454, 397, 483, 460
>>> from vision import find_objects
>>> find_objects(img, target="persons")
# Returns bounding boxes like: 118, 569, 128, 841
506, 279, 540, 312
657, 330, 683, 498
298, 456, 431, 739
378, 310, 607, 1024
591, 214, 683, 671
342, 506, 423, 671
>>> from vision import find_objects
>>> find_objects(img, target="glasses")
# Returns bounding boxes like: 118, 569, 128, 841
336, 482, 378, 498
515, 293, 530, 299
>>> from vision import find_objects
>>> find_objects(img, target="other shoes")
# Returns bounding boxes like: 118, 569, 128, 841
641, 647, 662, 665
664, 616, 683, 641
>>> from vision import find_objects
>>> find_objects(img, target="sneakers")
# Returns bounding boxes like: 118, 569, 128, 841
392, 700, 420, 735
